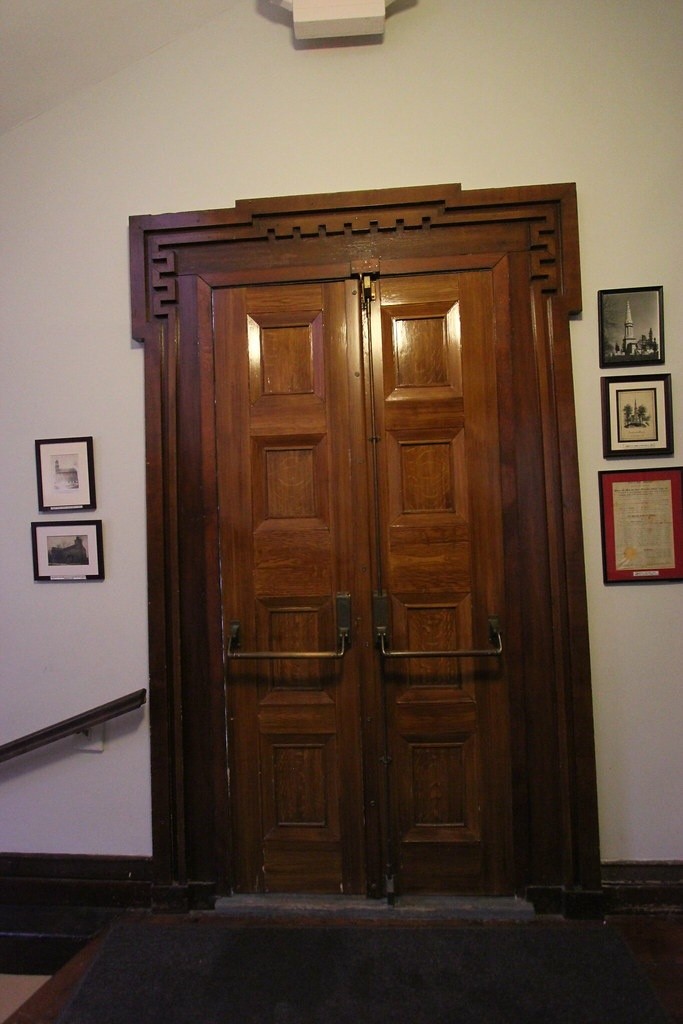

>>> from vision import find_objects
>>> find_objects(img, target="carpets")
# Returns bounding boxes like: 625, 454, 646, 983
56, 922, 670, 1023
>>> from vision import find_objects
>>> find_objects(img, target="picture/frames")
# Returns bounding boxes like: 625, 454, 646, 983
30, 519, 105, 581
596, 285, 666, 369
599, 373, 676, 459
35, 436, 97, 511
597, 466, 683, 585
